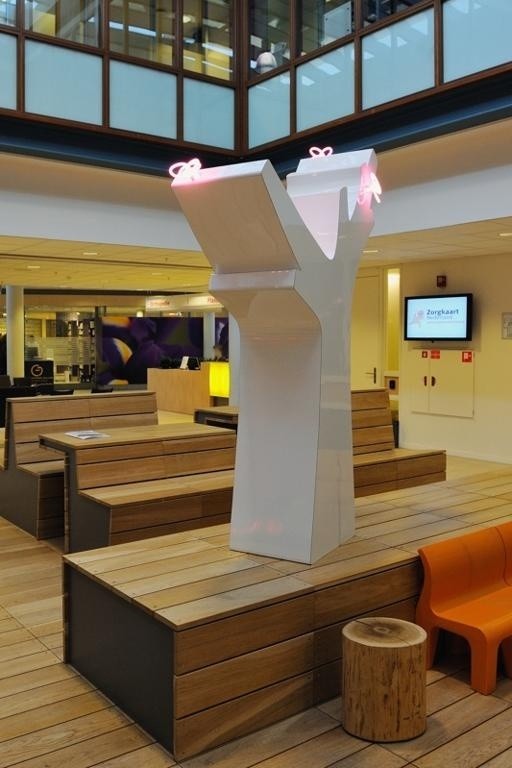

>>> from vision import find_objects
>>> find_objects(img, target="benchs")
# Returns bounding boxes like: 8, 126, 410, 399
34, 423, 236, 555
0, 390, 157, 541
351, 388, 446, 499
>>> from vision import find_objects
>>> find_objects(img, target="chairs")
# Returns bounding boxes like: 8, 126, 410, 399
414, 519, 511, 697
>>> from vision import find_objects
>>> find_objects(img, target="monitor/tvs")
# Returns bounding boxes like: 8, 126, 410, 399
91, 387, 114, 393
187, 356, 200, 370
404, 292, 472, 341
54, 389, 74, 395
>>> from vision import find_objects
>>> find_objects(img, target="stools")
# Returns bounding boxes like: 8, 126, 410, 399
341, 615, 427, 742
